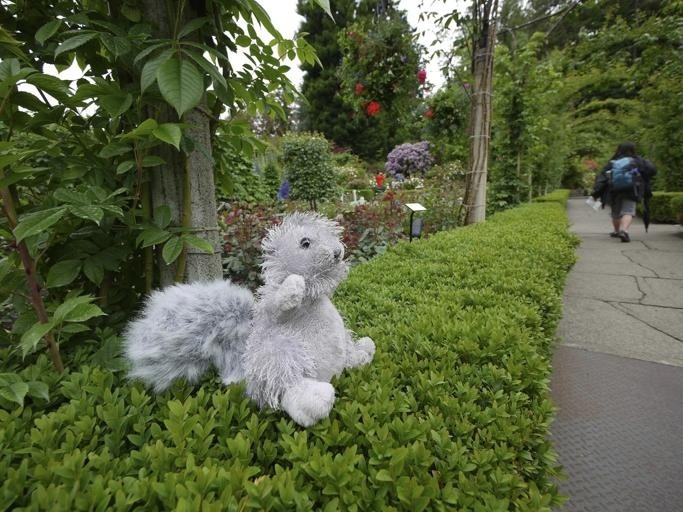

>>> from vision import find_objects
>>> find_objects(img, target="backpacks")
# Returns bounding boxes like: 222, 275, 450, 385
606, 157, 641, 192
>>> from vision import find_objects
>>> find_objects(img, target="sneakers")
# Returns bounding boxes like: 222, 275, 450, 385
610, 231, 630, 242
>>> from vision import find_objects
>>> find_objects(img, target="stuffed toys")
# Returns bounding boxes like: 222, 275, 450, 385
124, 212, 375, 428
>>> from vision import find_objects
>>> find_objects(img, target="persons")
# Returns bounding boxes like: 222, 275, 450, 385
592, 140, 657, 242
